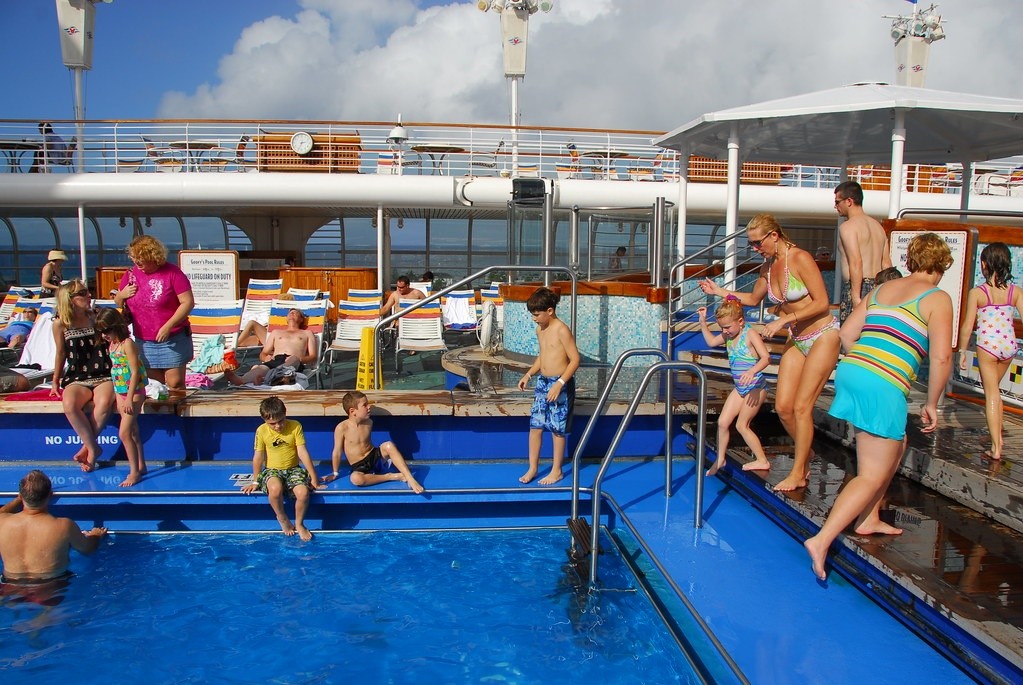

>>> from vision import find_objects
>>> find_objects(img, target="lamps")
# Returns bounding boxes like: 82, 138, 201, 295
390, 113, 408, 176
120, 217, 126, 227
146, 216, 152, 226
618, 222, 623, 232
398, 217, 403, 228
642, 223, 646, 232
372, 217, 376, 227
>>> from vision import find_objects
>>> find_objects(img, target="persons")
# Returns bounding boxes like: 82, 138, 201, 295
609, 247, 626, 274
804, 233, 954, 581
240, 395, 328, 542
49, 277, 149, 488
0, 469, 108, 606
224, 293, 317, 386
420, 271, 434, 282
380, 276, 426, 356
834, 181, 903, 356
38, 251, 68, 298
696, 293, 772, 476
0, 306, 38, 348
115, 234, 194, 391
697, 213, 842, 491
28, 123, 67, 174
518, 286, 581, 485
319, 391, 424, 494
959, 242, 1023, 459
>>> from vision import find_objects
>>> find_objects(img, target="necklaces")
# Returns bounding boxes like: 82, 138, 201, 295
110, 340, 121, 354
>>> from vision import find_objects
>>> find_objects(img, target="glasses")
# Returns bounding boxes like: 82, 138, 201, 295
69, 289, 87, 297
23, 311, 33, 313
747, 230, 774, 247
835, 197, 848, 204
98, 328, 111, 334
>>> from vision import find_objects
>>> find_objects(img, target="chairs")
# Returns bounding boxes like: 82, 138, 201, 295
781, 166, 812, 187
518, 142, 681, 184
140, 132, 250, 173
467, 141, 504, 177
0, 279, 503, 395
37, 136, 77, 173
388, 139, 422, 175
928, 163, 1023, 197
118, 159, 145, 173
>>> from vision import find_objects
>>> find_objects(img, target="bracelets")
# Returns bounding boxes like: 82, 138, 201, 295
331, 472, 339, 476
793, 312, 799, 333
252, 481, 259, 485
558, 378, 565, 386
526, 373, 532, 381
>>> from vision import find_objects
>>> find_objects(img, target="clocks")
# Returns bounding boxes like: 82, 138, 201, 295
291, 132, 313, 154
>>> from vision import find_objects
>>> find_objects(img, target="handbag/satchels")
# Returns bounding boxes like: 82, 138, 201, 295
122, 268, 133, 326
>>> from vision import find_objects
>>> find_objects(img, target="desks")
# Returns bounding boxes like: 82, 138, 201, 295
170, 141, 218, 172
412, 146, 465, 176
280, 266, 377, 325
0, 139, 40, 173
96, 267, 131, 299
586, 151, 628, 181
951, 167, 998, 186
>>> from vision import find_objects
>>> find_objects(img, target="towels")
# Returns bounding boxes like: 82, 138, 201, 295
184, 373, 215, 388
444, 296, 475, 328
189, 334, 226, 373
15, 311, 69, 370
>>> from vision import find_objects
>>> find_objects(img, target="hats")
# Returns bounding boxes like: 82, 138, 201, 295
48, 250, 68, 261
